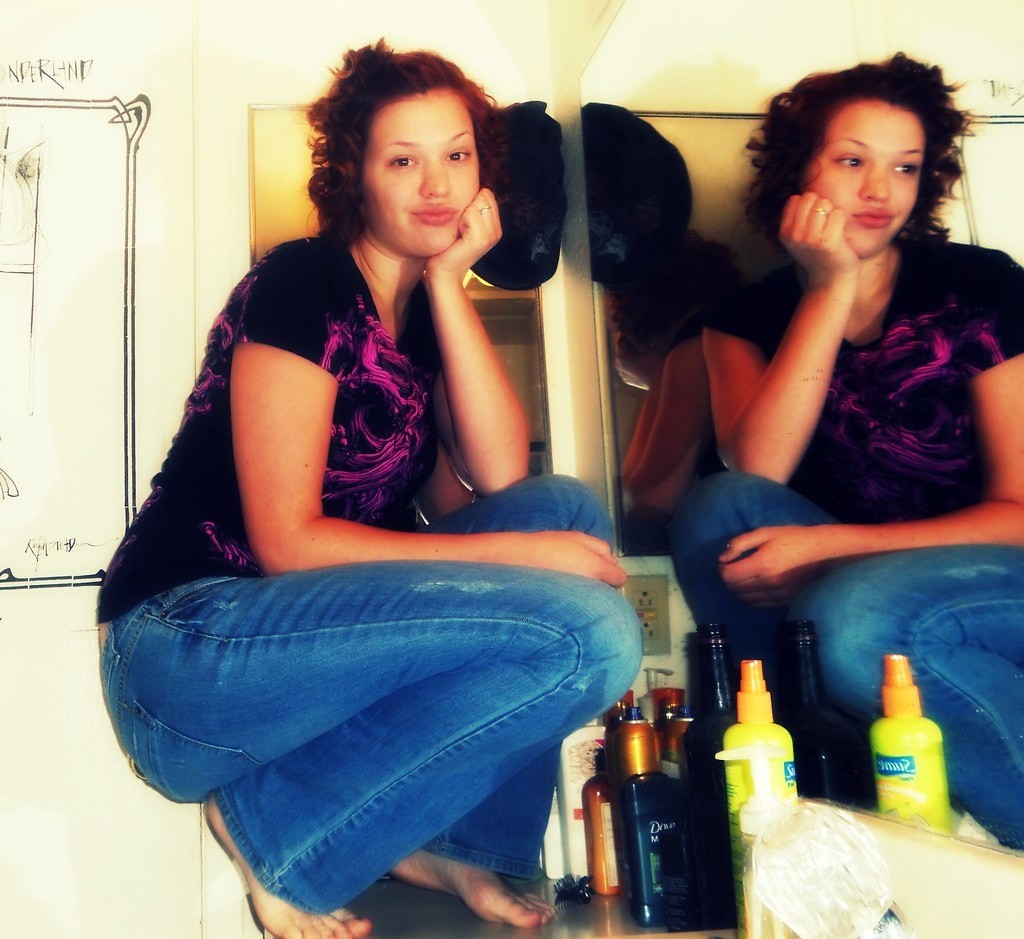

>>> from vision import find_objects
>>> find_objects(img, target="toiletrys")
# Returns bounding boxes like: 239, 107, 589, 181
687, 615, 962, 836
536, 659, 914, 939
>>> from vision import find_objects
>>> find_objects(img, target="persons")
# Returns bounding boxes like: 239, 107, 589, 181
112, 28, 647, 937
660, 50, 1023, 848
579, 102, 752, 550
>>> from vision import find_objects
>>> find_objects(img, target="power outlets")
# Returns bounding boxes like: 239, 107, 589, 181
619, 573, 674, 657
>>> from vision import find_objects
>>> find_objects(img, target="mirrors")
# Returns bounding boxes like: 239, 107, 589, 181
584, 0, 1024, 858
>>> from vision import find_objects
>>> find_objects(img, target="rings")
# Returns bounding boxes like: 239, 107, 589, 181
811, 205, 828, 219
478, 204, 492, 218
